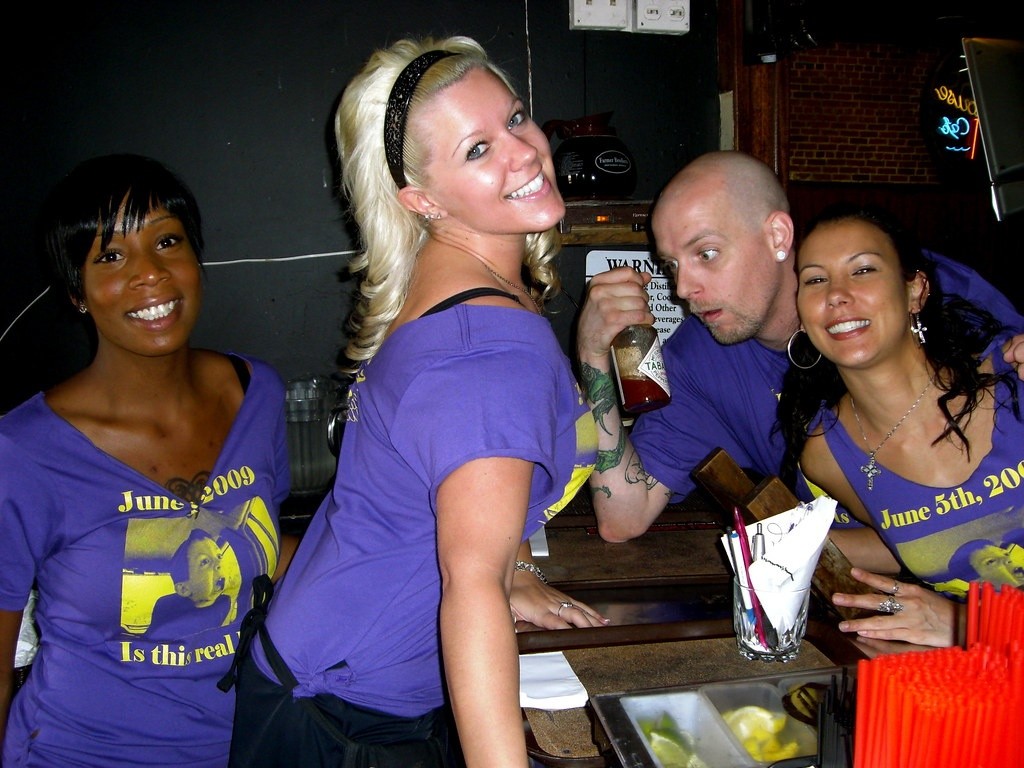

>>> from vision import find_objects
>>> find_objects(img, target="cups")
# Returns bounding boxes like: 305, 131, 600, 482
733, 576, 811, 664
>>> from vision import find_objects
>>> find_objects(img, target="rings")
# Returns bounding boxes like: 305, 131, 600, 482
890, 578, 900, 595
558, 601, 573, 617
874, 595, 903, 613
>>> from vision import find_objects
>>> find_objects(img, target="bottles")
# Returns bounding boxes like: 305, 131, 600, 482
608, 322, 673, 416
282, 376, 338, 497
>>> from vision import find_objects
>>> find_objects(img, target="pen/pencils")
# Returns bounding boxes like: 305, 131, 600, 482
721, 506, 768, 648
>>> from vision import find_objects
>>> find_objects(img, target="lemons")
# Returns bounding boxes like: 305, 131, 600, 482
639, 711, 705, 768
722, 707, 800, 762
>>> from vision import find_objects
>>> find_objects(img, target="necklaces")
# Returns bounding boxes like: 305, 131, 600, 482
485, 264, 544, 316
851, 371, 938, 491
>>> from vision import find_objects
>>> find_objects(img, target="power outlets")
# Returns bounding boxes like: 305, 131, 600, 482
569, 0, 627, 32
628, 0, 690, 35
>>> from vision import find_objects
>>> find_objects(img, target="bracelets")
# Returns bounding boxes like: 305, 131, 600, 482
513, 560, 548, 584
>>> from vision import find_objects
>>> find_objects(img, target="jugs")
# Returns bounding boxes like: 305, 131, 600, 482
537, 108, 637, 200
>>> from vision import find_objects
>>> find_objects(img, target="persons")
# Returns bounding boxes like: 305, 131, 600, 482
233, 36, 597, 768
0, 155, 301, 768
764, 199, 1024, 648
575, 150, 1024, 543
